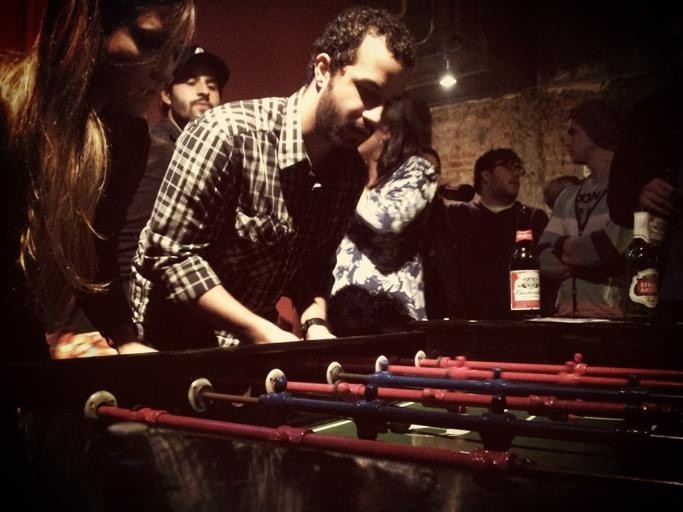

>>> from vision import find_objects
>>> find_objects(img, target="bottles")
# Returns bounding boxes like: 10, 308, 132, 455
507, 206, 542, 321
621, 207, 661, 323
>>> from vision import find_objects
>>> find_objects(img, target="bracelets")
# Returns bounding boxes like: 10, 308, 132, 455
298, 318, 326, 341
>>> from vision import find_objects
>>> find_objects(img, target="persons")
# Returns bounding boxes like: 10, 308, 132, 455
129, 4, 426, 349
63, 41, 234, 351
424, 146, 549, 317
321, 92, 440, 338
540, 175, 583, 211
536, 98, 634, 322
0, 1, 198, 412
607, 91, 683, 327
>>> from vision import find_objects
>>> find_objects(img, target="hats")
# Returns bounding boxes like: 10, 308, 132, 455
569, 100, 622, 150
172, 46, 230, 85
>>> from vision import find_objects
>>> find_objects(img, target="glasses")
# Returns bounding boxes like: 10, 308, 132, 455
494, 160, 525, 175
